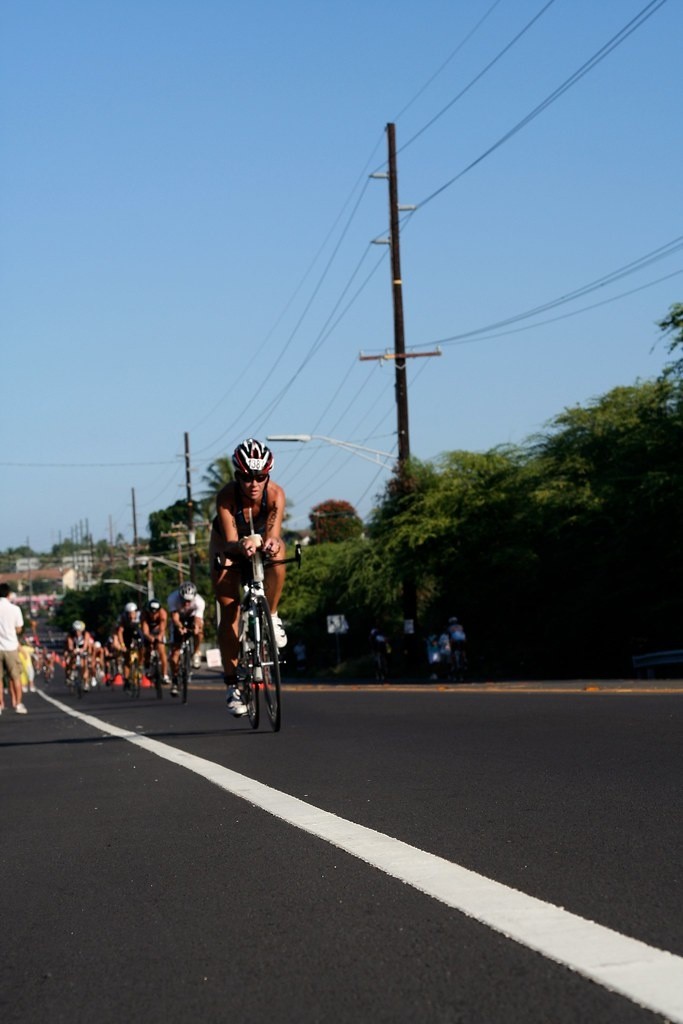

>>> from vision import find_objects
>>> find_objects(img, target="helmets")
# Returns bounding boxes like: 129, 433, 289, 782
147, 600, 162, 614
231, 437, 275, 476
449, 617, 458, 622
180, 581, 197, 602
72, 620, 85, 631
124, 602, 138, 612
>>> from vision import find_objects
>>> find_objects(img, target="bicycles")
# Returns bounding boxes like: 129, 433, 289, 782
210, 544, 303, 732
23, 612, 197, 704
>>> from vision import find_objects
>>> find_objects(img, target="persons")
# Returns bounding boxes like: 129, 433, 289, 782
2, 574, 207, 710
200, 441, 289, 711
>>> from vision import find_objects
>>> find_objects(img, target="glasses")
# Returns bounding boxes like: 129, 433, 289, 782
237, 471, 268, 482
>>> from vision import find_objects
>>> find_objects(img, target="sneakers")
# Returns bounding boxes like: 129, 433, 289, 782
225, 684, 248, 714
171, 683, 179, 697
192, 653, 202, 669
270, 617, 288, 648
16, 704, 28, 714
